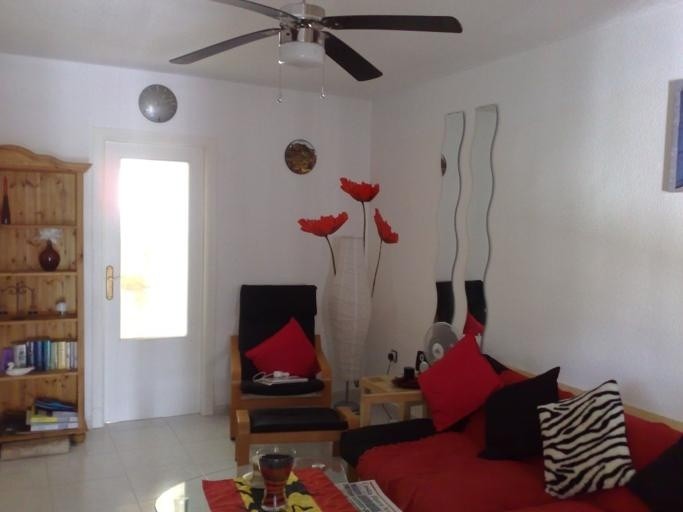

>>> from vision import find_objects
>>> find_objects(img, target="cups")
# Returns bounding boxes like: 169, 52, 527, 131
256, 445, 298, 512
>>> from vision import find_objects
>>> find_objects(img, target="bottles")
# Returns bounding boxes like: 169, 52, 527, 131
1, 194, 11, 224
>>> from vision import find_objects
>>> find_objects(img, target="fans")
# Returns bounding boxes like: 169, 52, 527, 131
168, 0, 462, 105
422, 322, 461, 369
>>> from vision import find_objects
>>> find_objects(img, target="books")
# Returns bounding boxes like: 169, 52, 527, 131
1, 338, 79, 436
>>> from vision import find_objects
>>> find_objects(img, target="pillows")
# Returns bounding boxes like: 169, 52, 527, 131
536, 378, 635, 501
244, 317, 322, 379
477, 366, 560, 460
414, 329, 503, 433
625, 436, 683, 512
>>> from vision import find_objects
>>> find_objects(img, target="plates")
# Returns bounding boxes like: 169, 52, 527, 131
241, 473, 296, 489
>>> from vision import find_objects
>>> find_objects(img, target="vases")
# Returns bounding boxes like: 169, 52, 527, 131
259, 453, 294, 512
320, 236, 372, 411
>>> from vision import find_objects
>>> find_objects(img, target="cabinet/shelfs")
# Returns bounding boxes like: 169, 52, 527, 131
0, 144, 93, 446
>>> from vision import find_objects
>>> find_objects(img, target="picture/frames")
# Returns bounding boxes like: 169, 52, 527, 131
662, 80, 683, 193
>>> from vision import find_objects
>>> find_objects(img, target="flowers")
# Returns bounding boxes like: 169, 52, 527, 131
297, 176, 400, 299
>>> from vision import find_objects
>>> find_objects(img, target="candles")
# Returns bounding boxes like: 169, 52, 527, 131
3, 176, 8, 195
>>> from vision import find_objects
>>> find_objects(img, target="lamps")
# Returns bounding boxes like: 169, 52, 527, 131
276, 28, 326, 102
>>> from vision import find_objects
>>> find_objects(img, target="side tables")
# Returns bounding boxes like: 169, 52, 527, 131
358, 376, 430, 422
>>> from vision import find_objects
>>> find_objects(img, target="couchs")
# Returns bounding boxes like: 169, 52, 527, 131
339, 354, 683, 511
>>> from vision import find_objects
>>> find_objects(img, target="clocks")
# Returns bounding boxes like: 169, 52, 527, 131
139, 85, 177, 123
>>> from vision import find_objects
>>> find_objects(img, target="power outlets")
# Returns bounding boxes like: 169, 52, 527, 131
390, 349, 398, 363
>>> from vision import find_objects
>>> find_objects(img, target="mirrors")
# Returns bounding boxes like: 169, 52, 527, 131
463, 104, 498, 355
433, 110, 464, 325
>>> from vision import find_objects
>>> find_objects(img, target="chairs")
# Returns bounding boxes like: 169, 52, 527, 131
231, 284, 359, 473
